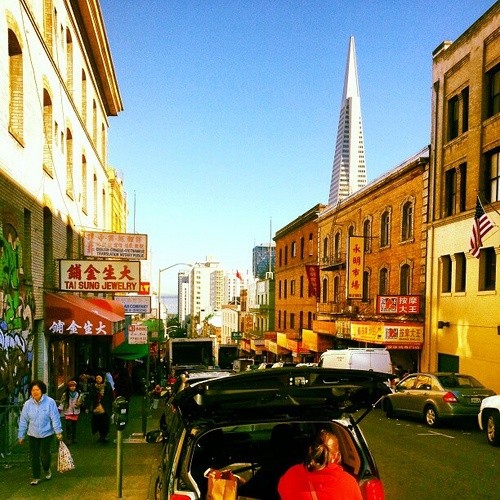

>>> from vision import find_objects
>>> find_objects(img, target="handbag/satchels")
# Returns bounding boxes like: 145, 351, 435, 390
57, 440, 75, 473
204, 468, 246, 500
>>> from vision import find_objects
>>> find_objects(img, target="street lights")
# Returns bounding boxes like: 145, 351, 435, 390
156, 263, 193, 387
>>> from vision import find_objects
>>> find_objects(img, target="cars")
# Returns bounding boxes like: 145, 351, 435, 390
380, 370, 495, 431
147, 366, 387, 500
159, 346, 393, 443
476, 394, 500, 445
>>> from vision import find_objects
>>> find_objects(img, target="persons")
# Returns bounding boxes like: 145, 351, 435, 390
58, 381, 82, 445
278, 430, 363, 500
84, 374, 113, 445
17, 381, 63, 485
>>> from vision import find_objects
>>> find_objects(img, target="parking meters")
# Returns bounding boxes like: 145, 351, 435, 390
110, 396, 129, 498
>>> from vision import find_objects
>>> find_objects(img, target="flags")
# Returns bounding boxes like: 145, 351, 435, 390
469, 195, 493, 259
236, 270, 242, 280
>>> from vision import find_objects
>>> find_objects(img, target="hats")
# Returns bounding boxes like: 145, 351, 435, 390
68, 381, 78, 387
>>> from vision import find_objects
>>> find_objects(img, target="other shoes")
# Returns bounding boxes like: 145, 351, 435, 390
30, 479, 41, 485
45, 468, 52, 479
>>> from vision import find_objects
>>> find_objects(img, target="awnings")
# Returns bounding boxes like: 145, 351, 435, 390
43, 291, 126, 335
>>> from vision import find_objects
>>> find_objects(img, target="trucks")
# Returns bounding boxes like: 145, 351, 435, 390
163, 333, 222, 381
